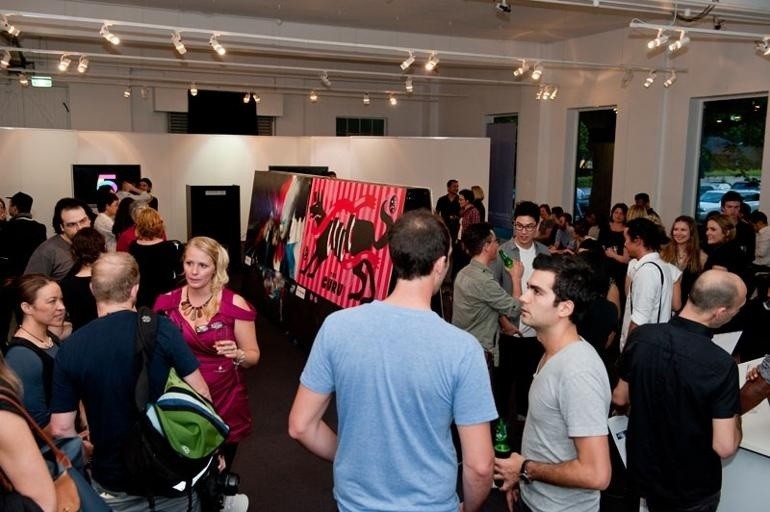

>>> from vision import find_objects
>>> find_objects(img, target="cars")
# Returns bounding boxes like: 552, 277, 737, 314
698, 180, 760, 223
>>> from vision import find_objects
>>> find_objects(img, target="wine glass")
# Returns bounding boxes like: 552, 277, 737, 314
213, 325, 229, 374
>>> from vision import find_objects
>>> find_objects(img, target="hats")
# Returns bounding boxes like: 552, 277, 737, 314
5, 191, 32, 208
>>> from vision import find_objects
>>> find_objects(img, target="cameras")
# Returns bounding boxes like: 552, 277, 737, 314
198, 457, 241, 512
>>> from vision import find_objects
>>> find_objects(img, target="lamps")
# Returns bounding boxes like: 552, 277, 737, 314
244, 90, 251, 103
58, 55, 71, 71
627, 13, 770, 59
400, 51, 415, 71
31, 75, 53, 88
190, 82, 198, 96
310, 91, 318, 103
123, 88, 131, 97
644, 68, 657, 89
531, 62, 544, 80
389, 93, 398, 106
253, 92, 261, 103
405, 76, 414, 93
664, 69, 676, 89
363, 94, 371, 104
514, 60, 530, 78
100, 23, 121, 45
77, 55, 89, 74
535, 83, 559, 100
0, 53, 12, 69
210, 33, 226, 56
622, 70, 634, 89
320, 71, 332, 87
171, 32, 187, 56
425, 51, 440, 73
19, 74, 29, 86
8, 27, 24, 39
141, 88, 148, 98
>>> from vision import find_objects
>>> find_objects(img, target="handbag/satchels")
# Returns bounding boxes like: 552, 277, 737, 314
125, 344, 230, 498
1, 467, 113, 512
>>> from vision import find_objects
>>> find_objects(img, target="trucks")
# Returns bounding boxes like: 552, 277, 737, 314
574, 187, 591, 220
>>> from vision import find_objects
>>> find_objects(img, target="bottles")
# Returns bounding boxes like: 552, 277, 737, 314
493, 417, 511, 486
499, 250, 514, 269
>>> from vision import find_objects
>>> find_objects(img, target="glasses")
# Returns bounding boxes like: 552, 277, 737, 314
515, 221, 538, 231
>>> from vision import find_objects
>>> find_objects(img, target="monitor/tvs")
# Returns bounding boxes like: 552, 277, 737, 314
71, 163, 143, 210
